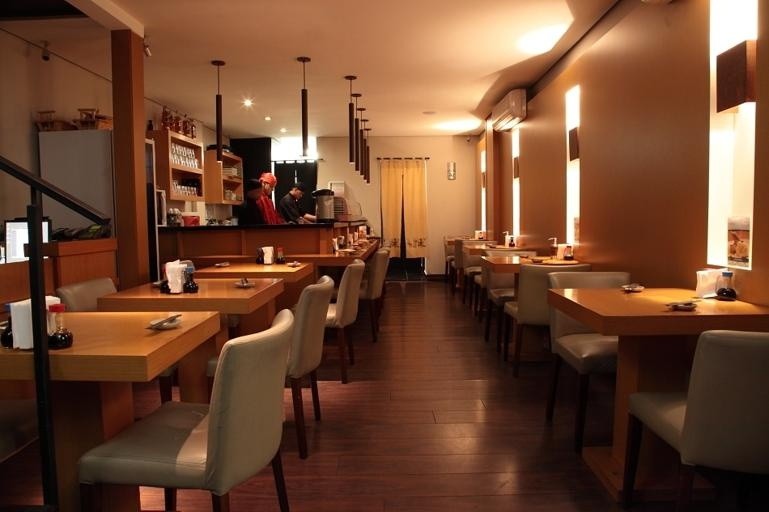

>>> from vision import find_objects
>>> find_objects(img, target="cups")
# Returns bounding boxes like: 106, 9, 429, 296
230, 217, 239, 225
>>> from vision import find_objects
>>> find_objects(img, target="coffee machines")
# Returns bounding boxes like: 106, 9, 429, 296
311, 189, 335, 223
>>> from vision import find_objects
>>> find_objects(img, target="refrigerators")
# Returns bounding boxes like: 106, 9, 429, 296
39, 132, 161, 284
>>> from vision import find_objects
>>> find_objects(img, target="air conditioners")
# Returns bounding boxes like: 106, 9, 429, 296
491, 88, 527, 133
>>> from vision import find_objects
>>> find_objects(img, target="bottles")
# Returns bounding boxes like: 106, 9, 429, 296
0, 303, 13, 350
339, 236, 344, 248
147, 120, 153, 130
478, 232, 483, 239
276, 248, 285, 264
256, 248, 265, 264
564, 246, 574, 260
716, 272, 736, 301
159, 265, 170, 294
183, 267, 198, 292
171, 142, 198, 168
161, 104, 197, 139
509, 237, 516, 247
46, 303, 72, 349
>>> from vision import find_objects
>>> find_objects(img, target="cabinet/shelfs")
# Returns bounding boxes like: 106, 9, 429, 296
146, 127, 206, 201
205, 150, 245, 206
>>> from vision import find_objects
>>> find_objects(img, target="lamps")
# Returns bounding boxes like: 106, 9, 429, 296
297, 56, 311, 156
25, 38, 50, 61
211, 60, 226, 162
144, 34, 152, 58
344, 75, 371, 185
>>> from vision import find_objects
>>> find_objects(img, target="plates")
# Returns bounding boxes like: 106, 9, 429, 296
490, 246, 496, 248
520, 256, 528, 258
235, 282, 255, 287
486, 244, 491, 246
621, 284, 644, 291
665, 301, 697, 312
150, 317, 182, 330
287, 262, 301, 266
336, 249, 355, 253
532, 259, 543, 263
215, 262, 230, 266
223, 167, 239, 176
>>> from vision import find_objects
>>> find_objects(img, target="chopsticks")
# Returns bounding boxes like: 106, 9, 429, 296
145, 314, 182, 329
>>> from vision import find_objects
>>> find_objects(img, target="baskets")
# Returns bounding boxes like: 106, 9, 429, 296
73, 108, 112, 129
35, 109, 77, 130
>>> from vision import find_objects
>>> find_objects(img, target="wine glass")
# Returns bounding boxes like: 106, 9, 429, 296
173, 185, 197, 197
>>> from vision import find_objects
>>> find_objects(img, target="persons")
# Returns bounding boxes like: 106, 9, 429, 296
239, 178, 265, 227
279, 182, 317, 224
256, 172, 287, 225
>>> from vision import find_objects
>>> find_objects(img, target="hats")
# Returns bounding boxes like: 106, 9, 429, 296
291, 182, 305, 189
259, 173, 277, 186
